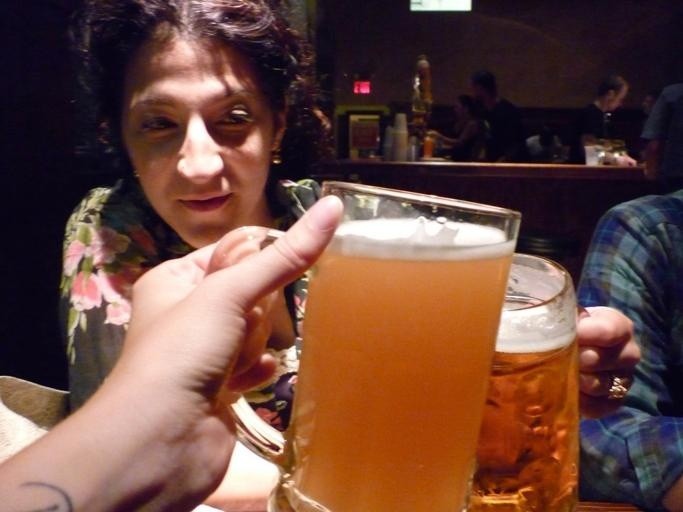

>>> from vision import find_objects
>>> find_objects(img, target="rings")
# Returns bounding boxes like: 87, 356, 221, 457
607, 375, 629, 401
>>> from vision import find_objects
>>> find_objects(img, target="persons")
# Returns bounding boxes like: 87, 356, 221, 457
554, 72, 639, 167
470, 67, 523, 161
54, 0, 643, 498
636, 75, 682, 180
559, 176, 683, 510
0, 191, 347, 509
424, 92, 488, 162
630, 89, 658, 136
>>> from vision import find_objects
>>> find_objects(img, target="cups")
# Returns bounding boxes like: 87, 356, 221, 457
466, 251, 592, 512
384, 113, 418, 161
423, 137, 433, 157
204, 179, 522, 512
